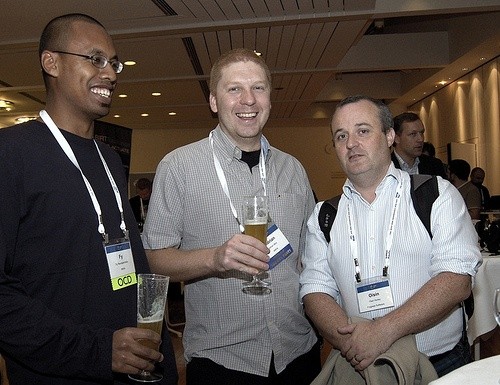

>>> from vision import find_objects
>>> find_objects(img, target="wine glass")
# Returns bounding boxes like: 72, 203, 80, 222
240, 197, 271, 285
128, 274, 170, 383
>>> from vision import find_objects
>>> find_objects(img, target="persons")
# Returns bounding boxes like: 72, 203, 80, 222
140, 46, 324, 385
300, 94, 483, 379
417, 142, 491, 223
0, 12, 179, 385
129, 178, 152, 233
383, 112, 447, 178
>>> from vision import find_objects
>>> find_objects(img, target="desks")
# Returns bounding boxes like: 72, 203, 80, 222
472, 221, 500, 361
429, 355, 500, 385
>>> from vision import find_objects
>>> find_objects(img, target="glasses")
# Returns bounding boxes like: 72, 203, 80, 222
48, 50, 125, 73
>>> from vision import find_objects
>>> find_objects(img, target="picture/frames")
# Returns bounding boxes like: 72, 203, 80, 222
127, 171, 158, 201
